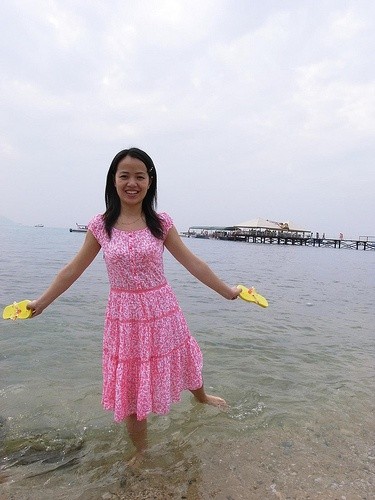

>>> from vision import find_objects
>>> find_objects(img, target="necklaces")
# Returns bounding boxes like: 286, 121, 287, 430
116, 214, 146, 225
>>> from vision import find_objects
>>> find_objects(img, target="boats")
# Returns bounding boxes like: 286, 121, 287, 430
35, 224, 44, 227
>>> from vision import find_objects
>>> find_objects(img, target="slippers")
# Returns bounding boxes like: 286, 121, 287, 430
3, 299, 33, 321
237, 284, 268, 308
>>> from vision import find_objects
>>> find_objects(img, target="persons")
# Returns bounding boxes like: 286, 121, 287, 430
27, 146, 241, 481
